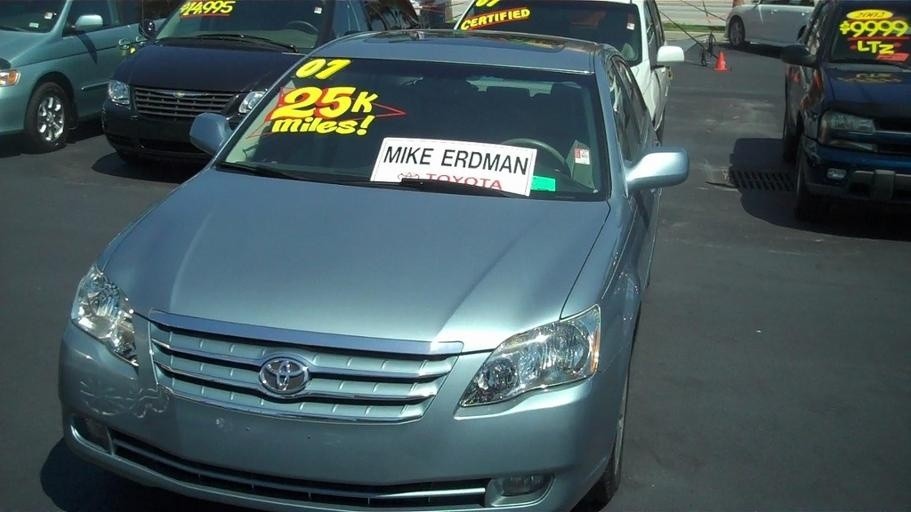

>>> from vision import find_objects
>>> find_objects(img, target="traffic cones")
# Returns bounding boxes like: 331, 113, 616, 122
714, 53, 729, 74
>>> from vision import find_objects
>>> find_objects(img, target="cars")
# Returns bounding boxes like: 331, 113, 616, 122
725, 0, 822, 51
783, 1, 911, 225
54, 24, 691, 512
